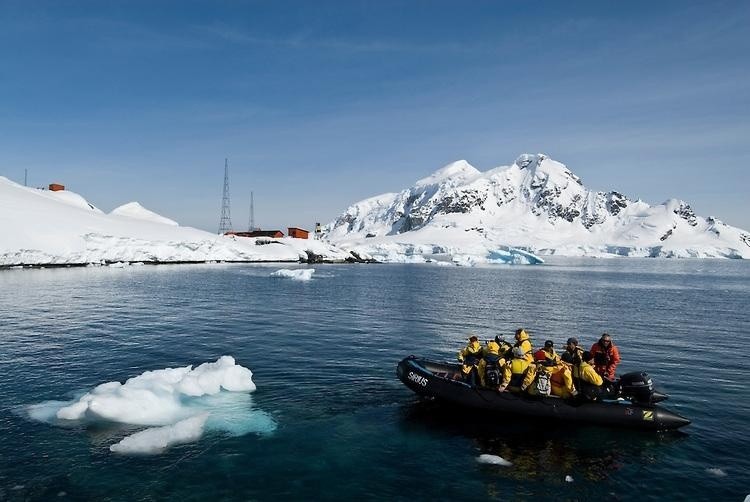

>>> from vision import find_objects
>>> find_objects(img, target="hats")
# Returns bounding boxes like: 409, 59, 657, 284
567, 337, 579, 345
582, 350, 595, 362
534, 350, 546, 361
544, 339, 554, 348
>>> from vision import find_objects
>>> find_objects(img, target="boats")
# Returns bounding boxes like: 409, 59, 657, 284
396, 355, 691, 431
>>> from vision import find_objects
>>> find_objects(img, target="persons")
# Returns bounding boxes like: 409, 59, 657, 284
453, 327, 621, 404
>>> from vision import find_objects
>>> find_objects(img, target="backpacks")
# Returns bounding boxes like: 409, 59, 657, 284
533, 369, 551, 398
483, 356, 502, 388
